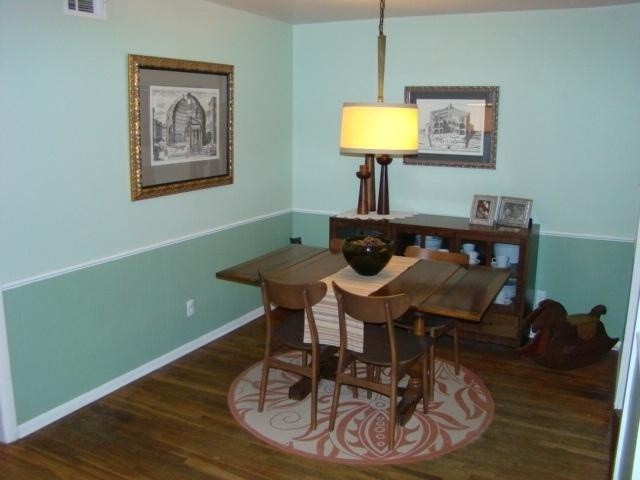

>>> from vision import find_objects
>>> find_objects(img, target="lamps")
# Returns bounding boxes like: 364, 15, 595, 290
339, 0, 419, 154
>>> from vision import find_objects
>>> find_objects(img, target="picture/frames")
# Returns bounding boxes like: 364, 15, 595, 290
469, 194, 497, 227
404, 86, 499, 169
127, 53, 234, 201
494, 195, 533, 229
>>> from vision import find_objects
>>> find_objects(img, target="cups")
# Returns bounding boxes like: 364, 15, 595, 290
493, 279, 517, 305
463, 243, 481, 265
492, 256, 509, 266
415, 236, 442, 251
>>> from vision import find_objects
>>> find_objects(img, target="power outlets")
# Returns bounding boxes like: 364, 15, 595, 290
186, 298, 194, 316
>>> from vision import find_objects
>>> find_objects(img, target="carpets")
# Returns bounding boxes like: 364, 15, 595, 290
227, 351, 494, 465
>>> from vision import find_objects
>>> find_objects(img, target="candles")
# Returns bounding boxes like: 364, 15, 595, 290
360, 165, 368, 175
381, 154, 389, 160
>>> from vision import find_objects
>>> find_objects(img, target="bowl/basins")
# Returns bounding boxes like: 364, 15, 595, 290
343, 235, 395, 277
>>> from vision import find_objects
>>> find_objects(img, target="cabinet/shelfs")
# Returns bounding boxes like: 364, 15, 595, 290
329, 210, 531, 351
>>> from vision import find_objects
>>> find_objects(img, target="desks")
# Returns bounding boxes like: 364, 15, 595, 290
215, 244, 512, 427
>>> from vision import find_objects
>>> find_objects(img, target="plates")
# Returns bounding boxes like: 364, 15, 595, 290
491, 262, 510, 269
